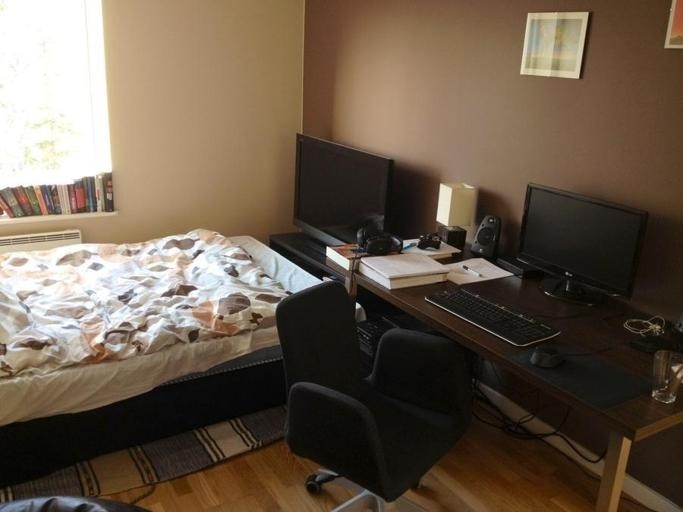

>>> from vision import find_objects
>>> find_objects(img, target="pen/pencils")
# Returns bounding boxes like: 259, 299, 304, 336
462, 265, 481, 277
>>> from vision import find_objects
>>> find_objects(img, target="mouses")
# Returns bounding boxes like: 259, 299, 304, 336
529, 345, 575, 369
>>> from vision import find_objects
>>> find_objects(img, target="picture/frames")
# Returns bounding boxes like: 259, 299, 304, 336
519, 10, 590, 82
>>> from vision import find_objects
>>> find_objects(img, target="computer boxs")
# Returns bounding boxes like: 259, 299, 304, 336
354, 312, 437, 375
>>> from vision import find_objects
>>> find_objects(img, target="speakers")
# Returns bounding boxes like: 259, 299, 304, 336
470, 215, 502, 255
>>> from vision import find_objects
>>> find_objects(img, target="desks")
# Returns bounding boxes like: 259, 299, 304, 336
269, 232, 329, 276
325, 238, 683, 511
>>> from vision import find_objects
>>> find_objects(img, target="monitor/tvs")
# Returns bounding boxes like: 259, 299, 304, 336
517, 183, 649, 302
291, 132, 396, 247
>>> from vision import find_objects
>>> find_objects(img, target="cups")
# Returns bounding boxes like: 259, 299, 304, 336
651, 350, 683, 404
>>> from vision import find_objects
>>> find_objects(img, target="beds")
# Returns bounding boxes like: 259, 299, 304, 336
0, 228, 367, 484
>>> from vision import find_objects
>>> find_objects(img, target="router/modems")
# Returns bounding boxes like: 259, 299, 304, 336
495, 256, 545, 278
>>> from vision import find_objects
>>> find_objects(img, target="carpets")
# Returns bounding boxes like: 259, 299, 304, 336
0, 404, 286, 505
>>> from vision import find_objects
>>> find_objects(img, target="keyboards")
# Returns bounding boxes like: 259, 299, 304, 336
424, 286, 563, 346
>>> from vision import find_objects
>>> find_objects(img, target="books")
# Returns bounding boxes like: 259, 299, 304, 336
325, 243, 400, 271
358, 263, 447, 291
0, 172, 113, 219
360, 252, 449, 277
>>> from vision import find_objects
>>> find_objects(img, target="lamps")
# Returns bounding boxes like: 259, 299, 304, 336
436, 182, 478, 248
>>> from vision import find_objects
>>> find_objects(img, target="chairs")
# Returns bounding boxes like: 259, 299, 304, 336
275, 280, 471, 512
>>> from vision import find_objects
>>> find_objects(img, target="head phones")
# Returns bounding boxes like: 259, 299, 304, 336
357, 226, 404, 256
